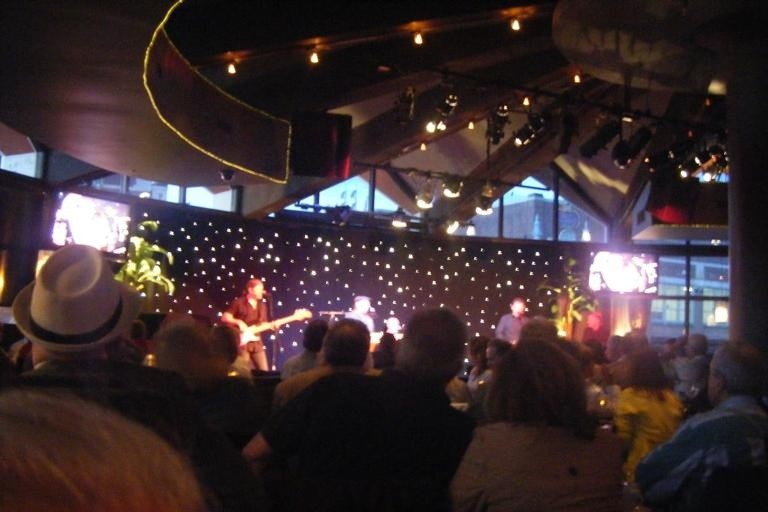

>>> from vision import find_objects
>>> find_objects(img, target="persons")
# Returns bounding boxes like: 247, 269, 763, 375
1, 243, 767, 510
49, 195, 128, 252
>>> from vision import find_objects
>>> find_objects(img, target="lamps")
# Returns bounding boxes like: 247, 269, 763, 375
390, 175, 494, 227
435, 71, 723, 179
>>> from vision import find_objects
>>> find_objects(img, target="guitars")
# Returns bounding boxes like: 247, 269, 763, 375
239, 308, 312, 347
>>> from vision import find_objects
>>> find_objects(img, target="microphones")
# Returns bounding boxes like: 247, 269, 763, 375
263, 292, 273, 299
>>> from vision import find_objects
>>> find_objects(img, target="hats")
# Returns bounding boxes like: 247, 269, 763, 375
13, 244, 144, 353
154, 311, 208, 374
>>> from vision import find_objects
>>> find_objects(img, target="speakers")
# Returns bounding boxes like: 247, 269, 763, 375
290, 109, 352, 179
630, 180, 729, 242
